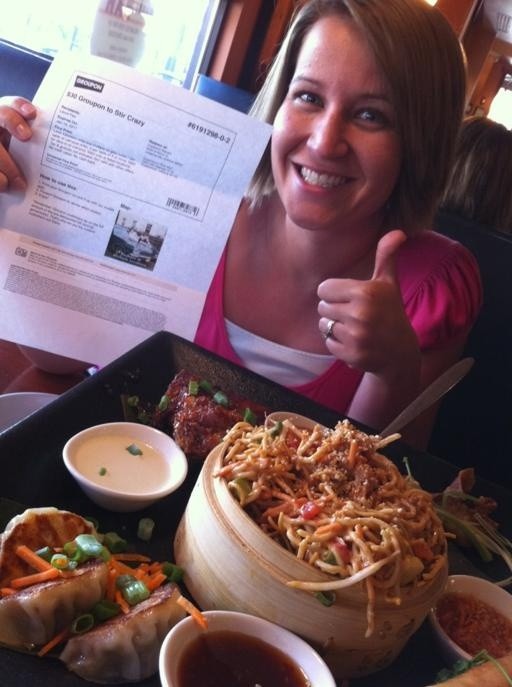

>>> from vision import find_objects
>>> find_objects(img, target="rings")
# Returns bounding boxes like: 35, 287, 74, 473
326, 319, 336, 336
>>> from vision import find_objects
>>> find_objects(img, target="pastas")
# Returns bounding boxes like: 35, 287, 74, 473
214, 420, 458, 637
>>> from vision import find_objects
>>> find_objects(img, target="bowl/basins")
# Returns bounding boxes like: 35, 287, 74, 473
429, 577, 512, 668
62, 422, 190, 510
157, 611, 336, 687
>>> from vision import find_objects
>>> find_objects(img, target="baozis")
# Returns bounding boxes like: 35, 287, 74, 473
0, 503, 188, 685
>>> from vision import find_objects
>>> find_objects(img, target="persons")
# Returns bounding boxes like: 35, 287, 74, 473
434, 113, 509, 235
0, 0, 490, 456
